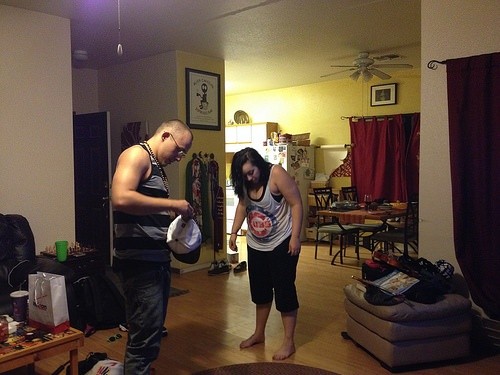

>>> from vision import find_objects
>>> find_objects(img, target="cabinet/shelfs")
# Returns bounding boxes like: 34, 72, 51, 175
225, 122, 277, 153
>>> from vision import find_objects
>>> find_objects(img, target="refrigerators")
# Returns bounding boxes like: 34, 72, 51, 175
258, 145, 315, 242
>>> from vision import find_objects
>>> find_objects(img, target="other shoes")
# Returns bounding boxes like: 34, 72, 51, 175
208, 261, 230, 276
234, 261, 247, 273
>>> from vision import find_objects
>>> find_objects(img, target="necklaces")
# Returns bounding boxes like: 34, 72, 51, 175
143, 139, 168, 193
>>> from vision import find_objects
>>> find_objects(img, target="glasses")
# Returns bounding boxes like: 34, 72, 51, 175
165, 131, 186, 158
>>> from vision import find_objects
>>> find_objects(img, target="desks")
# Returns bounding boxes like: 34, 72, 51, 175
316, 204, 417, 219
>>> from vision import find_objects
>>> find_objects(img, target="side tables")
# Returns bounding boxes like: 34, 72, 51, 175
41, 247, 100, 328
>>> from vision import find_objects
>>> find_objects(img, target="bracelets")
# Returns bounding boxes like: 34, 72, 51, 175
230, 232, 237, 235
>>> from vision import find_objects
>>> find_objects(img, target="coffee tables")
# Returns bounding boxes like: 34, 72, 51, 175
0, 317, 84, 375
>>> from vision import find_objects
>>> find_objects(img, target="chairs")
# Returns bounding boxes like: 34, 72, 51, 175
371, 192, 419, 255
340, 187, 387, 256
313, 186, 360, 265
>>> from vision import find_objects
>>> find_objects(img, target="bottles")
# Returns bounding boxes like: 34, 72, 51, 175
0, 315, 10, 344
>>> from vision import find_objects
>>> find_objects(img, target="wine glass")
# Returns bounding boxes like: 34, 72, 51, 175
364, 194, 372, 214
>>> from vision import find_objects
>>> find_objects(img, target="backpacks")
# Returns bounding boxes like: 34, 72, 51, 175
71, 267, 125, 328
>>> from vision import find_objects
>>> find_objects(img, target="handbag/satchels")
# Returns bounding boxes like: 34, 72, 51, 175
363, 251, 444, 305
28, 271, 69, 327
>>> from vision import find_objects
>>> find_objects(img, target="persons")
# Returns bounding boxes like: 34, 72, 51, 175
228, 148, 303, 360
111, 119, 194, 375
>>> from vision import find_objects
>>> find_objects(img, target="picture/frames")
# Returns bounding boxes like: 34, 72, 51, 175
185, 68, 221, 130
371, 83, 397, 106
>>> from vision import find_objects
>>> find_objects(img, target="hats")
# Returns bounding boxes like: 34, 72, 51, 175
166, 215, 201, 265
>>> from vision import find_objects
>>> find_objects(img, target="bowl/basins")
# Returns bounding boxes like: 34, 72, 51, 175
390, 203, 407, 210
378, 206, 393, 210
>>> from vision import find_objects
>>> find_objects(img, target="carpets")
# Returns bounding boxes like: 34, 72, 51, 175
193, 362, 340, 375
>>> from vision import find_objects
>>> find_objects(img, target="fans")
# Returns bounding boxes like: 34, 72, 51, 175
321, 51, 413, 83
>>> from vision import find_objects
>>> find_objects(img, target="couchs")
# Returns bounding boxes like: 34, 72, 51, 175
0, 212, 91, 332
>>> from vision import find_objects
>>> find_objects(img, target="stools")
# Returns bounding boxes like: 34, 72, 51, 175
344, 284, 472, 370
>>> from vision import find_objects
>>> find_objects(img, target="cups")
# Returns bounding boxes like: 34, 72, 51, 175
55, 240, 68, 262
10, 291, 30, 323
